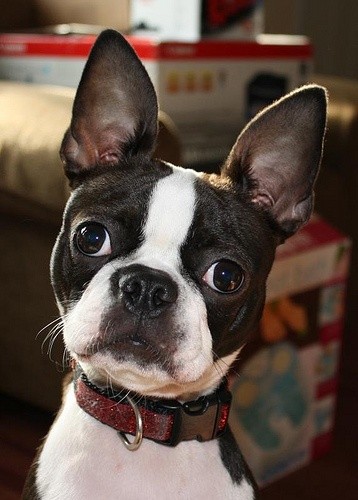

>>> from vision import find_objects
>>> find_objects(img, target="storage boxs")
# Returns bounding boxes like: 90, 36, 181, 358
215, 211, 357, 489
131, 0, 265, 41
0, 33, 314, 139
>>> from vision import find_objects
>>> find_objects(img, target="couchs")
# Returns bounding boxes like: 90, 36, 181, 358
0, 0, 358, 411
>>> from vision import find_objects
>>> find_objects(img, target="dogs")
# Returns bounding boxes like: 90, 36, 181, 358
20, 27, 329, 500
